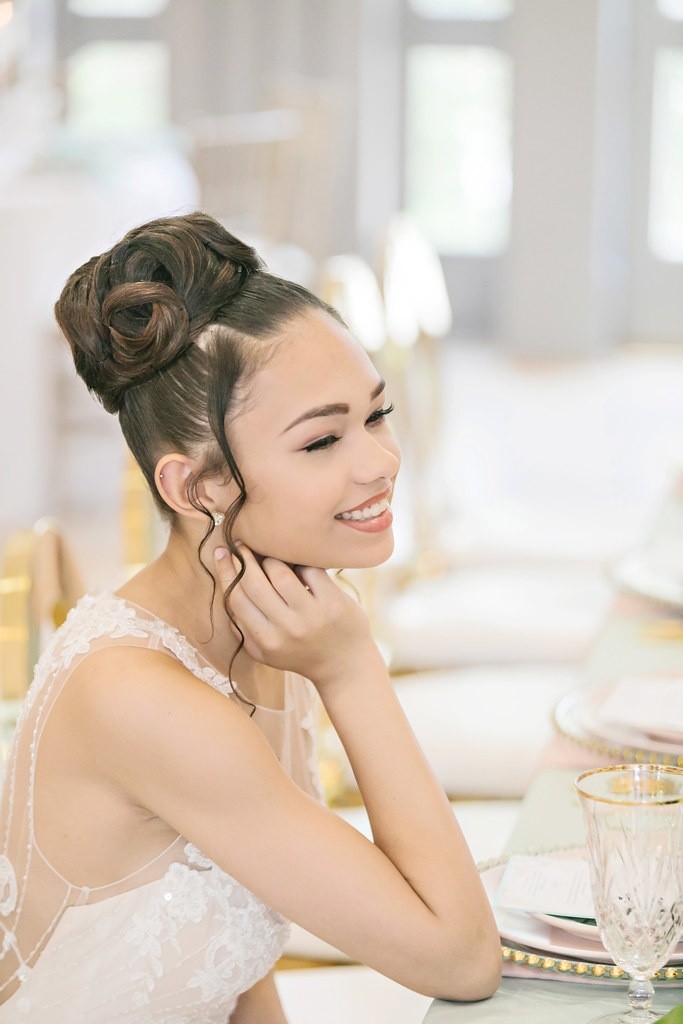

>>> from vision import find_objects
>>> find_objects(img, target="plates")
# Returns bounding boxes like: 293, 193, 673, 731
476, 572, 683, 982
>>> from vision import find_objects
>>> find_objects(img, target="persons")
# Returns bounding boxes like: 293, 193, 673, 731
0, 213, 503, 1024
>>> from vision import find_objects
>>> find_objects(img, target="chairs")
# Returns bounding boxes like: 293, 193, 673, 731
0, 229, 653, 1024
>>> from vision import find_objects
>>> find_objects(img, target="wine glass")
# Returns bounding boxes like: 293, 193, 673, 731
575, 766, 683, 1024
